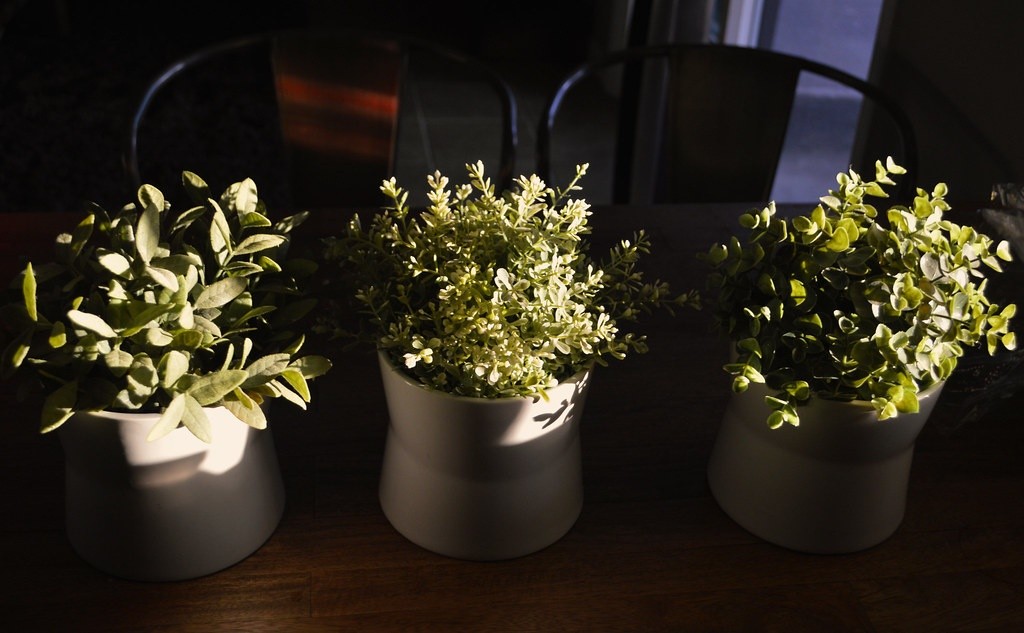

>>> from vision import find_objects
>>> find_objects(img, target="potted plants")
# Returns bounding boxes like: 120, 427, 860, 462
5, 170, 334, 585
705, 153, 1017, 558
324, 158, 707, 565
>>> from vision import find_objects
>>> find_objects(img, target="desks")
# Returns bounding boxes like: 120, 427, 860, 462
0, 201, 1024, 633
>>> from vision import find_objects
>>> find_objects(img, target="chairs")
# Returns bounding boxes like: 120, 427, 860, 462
537, 39, 920, 204
127, 21, 519, 206
